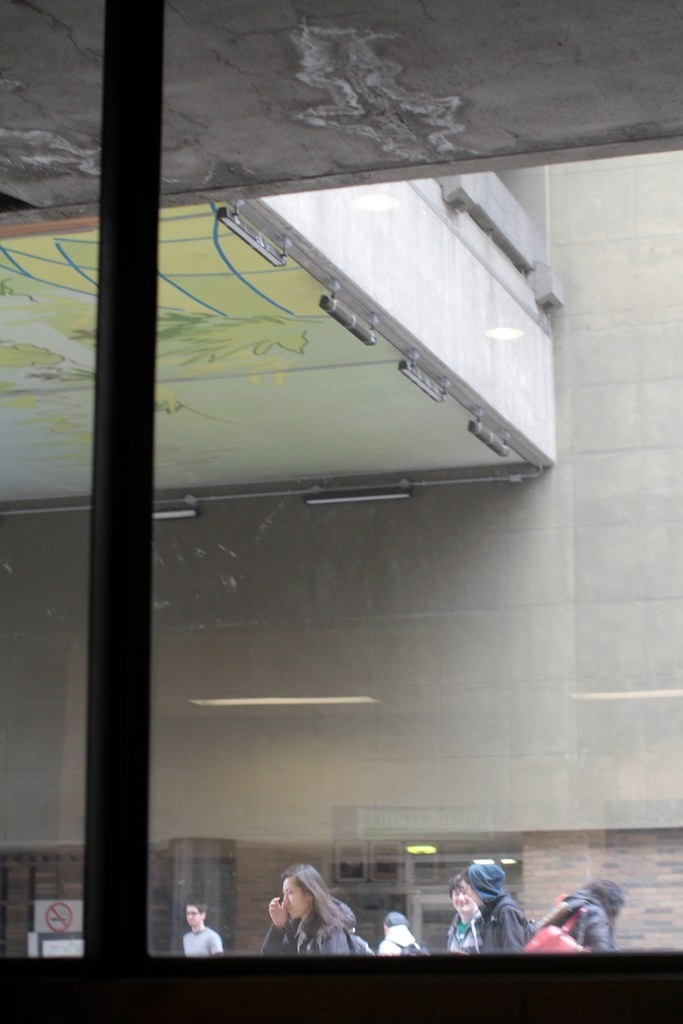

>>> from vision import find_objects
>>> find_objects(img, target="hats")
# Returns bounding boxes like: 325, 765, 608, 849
384, 911, 409, 930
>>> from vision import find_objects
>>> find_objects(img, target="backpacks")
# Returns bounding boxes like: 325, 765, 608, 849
387, 939, 431, 955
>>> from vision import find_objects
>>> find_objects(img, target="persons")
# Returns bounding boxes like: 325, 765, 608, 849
376, 911, 419, 957
181, 901, 223, 958
564, 880, 624, 952
443, 864, 529, 953
259, 865, 355, 956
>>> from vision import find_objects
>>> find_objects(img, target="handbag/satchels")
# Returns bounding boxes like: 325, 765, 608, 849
522, 906, 591, 953
